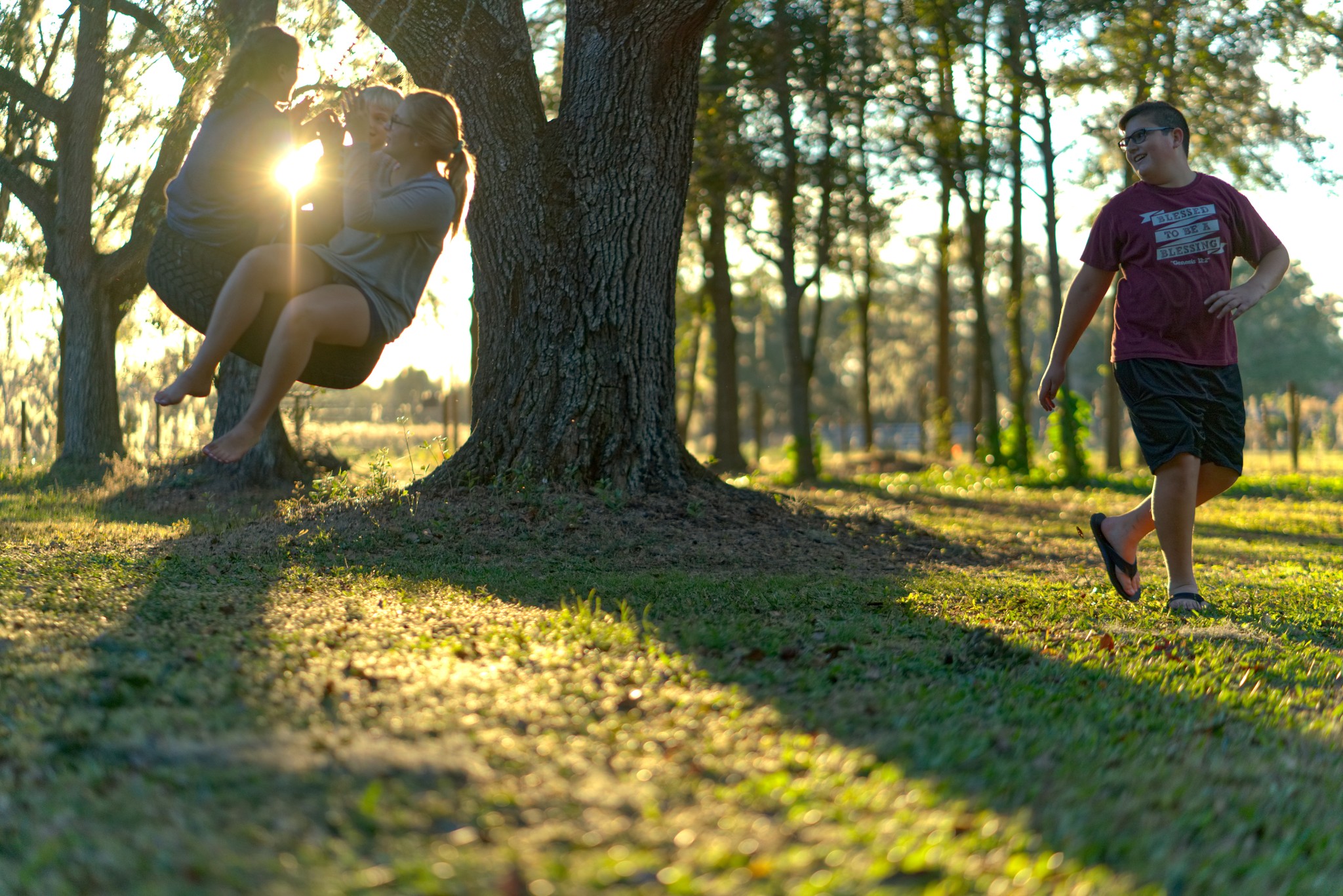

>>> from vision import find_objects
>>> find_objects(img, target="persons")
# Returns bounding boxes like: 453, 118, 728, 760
319, 86, 403, 211
162, 26, 333, 251
154, 91, 471, 462
1038, 102, 1290, 613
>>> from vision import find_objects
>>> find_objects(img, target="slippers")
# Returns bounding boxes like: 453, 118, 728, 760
1162, 590, 1223, 620
1089, 512, 1144, 603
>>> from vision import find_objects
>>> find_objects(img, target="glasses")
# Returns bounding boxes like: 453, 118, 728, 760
1117, 124, 1175, 153
283, 62, 304, 74
388, 114, 417, 132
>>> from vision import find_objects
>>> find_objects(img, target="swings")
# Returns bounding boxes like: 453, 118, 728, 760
143, 1, 472, 389
